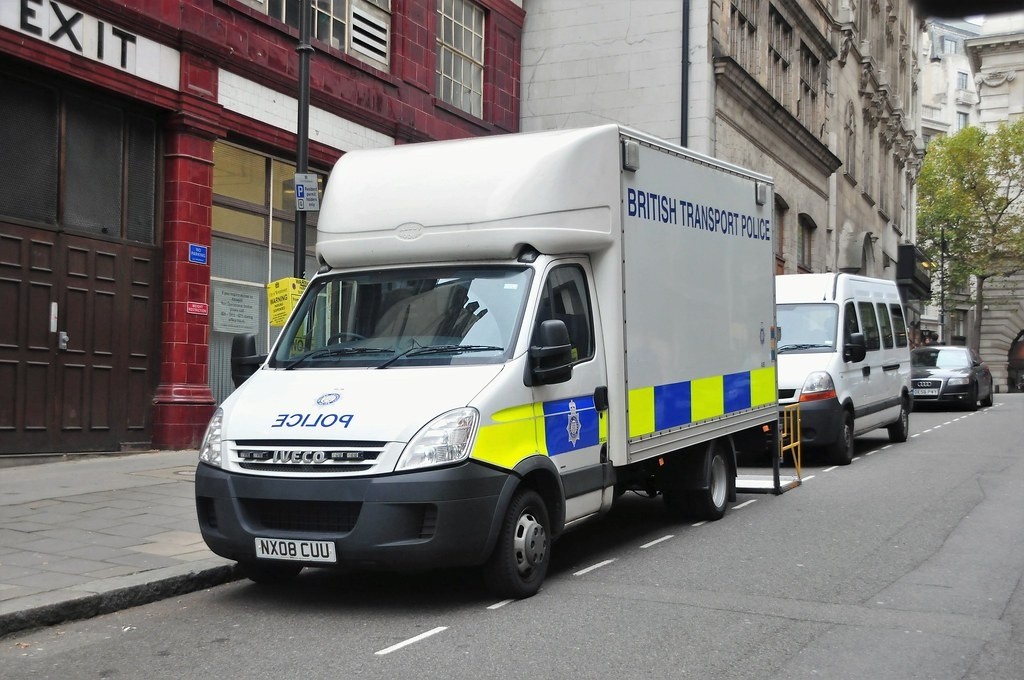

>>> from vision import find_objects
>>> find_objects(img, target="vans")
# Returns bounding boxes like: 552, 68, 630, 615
733, 273, 913, 466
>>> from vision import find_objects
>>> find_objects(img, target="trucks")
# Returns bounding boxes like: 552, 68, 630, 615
195, 128, 779, 603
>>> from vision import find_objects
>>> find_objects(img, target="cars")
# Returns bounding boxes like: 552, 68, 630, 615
912, 346, 992, 414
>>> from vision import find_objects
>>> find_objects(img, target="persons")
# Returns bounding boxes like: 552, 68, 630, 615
920, 337, 930, 346
927, 333, 939, 345
